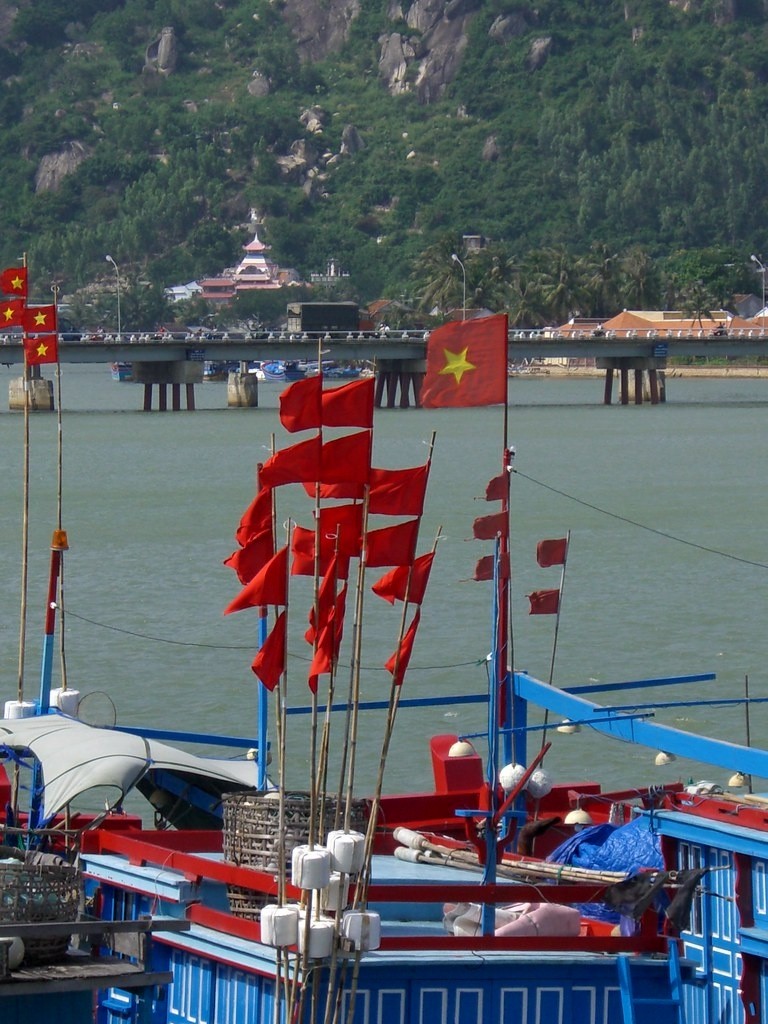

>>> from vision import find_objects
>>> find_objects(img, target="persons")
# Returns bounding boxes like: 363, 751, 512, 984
597, 322, 603, 335
716, 322, 727, 335
425, 331, 431, 335
94, 327, 104, 340
159, 325, 164, 335
196, 329, 201, 337
379, 324, 390, 338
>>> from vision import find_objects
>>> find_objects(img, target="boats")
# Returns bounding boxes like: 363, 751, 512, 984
0, 530, 768, 1024
111, 360, 362, 383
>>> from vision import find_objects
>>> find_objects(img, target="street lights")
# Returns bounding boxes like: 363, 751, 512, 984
751, 255, 764, 328
452, 254, 465, 320
106, 255, 120, 337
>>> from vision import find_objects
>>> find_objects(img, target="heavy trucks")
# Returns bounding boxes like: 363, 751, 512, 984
287, 301, 375, 337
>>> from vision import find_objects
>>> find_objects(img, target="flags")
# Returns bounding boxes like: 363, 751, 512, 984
368, 462, 430, 516
323, 378, 377, 428
473, 473, 509, 581
24, 334, 57, 366
420, 314, 508, 407
0, 267, 27, 297
278, 373, 324, 432
361, 519, 418, 567
23, 305, 56, 333
259, 433, 321, 490
301, 430, 371, 499
0, 299, 26, 328
224, 487, 434, 694
528, 539, 567, 615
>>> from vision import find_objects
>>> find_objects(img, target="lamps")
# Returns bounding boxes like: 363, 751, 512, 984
729, 772, 750, 788
558, 716, 581, 734
448, 739, 473, 757
564, 796, 593, 826
654, 751, 675, 766
